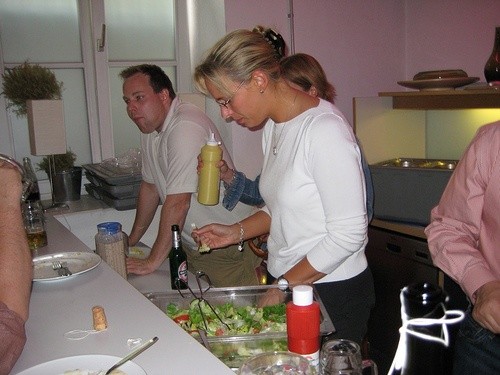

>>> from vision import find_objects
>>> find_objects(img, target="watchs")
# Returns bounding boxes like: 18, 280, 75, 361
278, 276, 292, 300
224, 168, 236, 189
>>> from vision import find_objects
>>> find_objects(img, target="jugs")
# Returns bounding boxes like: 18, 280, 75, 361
94, 222, 130, 282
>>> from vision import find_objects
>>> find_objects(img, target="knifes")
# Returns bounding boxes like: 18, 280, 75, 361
62, 262, 68, 277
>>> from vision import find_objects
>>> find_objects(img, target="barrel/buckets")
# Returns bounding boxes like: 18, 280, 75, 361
51, 167, 82, 201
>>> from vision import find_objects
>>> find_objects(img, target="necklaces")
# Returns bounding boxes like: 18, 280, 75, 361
272, 92, 298, 154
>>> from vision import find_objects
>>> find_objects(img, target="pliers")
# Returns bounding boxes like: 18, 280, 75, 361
175, 270, 231, 331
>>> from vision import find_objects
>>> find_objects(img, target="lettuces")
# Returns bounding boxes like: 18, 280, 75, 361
165, 300, 289, 364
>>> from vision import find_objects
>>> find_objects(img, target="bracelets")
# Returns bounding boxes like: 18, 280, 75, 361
237, 222, 244, 251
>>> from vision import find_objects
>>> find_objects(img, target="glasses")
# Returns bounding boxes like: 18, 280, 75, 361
215, 80, 246, 108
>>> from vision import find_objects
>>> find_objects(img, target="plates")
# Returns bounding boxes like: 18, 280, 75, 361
16, 355, 146, 375
394, 76, 480, 92
31, 252, 100, 283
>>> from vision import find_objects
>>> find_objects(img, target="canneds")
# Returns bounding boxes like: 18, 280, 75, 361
95, 222, 127, 279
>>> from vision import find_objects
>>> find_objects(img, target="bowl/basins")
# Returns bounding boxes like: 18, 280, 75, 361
144, 282, 336, 368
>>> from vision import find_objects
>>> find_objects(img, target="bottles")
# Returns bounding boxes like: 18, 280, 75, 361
367, 283, 458, 374
484, 27, 500, 85
169, 225, 189, 291
196, 132, 223, 206
286, 285, 321, 375
22, 157, 41, 200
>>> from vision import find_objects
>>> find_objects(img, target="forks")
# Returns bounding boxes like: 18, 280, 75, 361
52, 261, 63, 277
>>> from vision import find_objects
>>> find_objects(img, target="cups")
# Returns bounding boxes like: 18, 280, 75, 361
239, 351, 317, 375
319, 338, 377, 375
102, 151, 142, 174
20, 200, 48, 248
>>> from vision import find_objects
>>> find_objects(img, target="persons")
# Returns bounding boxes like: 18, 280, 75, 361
199, 53, 373, 225
118, 64, 263, 295
424, 119, 500, 375
190, 24, 374, 349
0, 152, 33, 375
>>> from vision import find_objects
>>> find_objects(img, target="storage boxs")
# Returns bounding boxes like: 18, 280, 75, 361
142, 284, 334, 367
367, 157, 460, 225
